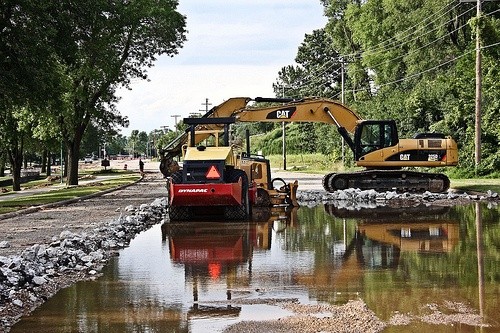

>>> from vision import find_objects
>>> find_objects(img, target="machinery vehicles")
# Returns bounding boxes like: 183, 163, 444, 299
181, 129, 300, 208
165, 117, 258, 223
159, 96, 459, 194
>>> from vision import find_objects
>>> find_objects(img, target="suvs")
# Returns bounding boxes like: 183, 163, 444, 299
85, 156, 92, 164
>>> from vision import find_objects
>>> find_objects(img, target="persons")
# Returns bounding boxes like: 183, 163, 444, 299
139, 159, 145, 176
385, 130, 391, 146
